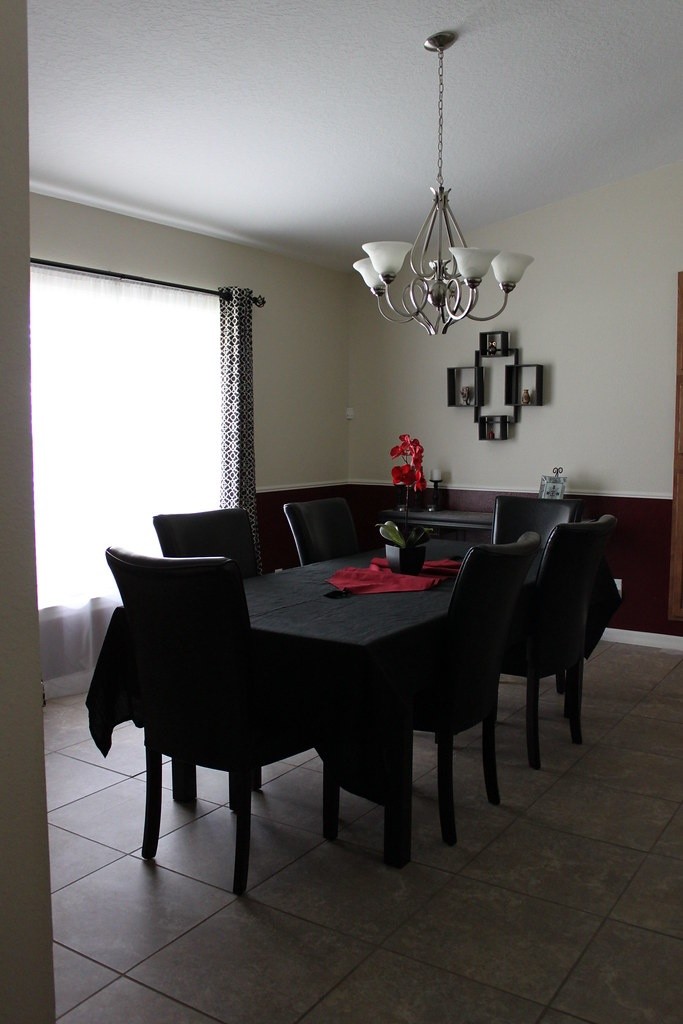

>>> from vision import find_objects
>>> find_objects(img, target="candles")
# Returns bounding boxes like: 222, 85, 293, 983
430, 469, 441, 480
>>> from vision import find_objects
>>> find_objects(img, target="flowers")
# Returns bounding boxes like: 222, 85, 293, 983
374, 434, 437, 548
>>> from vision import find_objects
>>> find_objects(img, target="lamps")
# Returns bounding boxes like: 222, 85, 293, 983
348, 30, 538, 335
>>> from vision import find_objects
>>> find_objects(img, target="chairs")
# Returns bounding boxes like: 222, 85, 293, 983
105, 545, 326, 898
314, 530, 543, 847
282, 495, 360, 568
500, 513, 618, 770
151, 507, 258, 579
490, 494, 585, 552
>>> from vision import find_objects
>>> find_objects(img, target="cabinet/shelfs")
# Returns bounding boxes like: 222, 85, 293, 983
446, 331, 543, 441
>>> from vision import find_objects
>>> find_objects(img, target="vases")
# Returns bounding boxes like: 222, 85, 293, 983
385, 543, 426, 575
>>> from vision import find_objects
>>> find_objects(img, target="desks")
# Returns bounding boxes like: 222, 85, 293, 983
380, 508, 493, 532
85, 536, 543, 867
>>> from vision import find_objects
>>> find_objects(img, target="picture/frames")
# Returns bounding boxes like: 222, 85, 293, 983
538, 467, 568, 500
